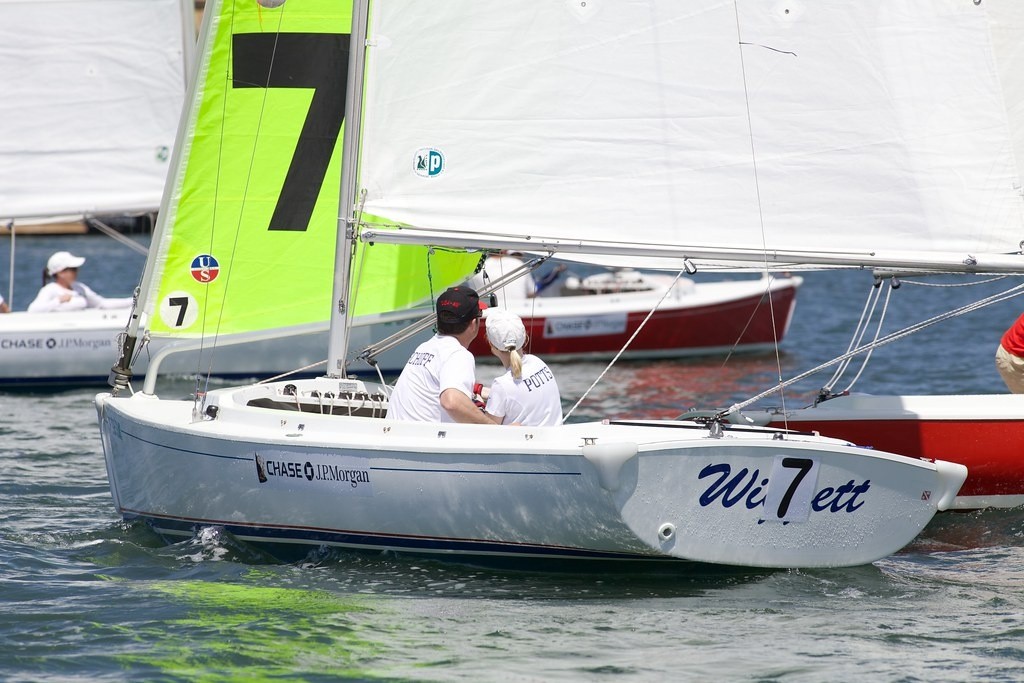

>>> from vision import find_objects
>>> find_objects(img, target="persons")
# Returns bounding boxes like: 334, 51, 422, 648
476, 248, 566, 299
995, 314, 1024, 394
485, 313, 562, 426
27, 252, 133, 312
0, 295, 9, 313
384, 286, 522, 426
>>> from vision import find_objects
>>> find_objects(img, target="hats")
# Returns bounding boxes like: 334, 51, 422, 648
485, 307, 526, 352
46, 251, 85, 277
437, 286, 488, 323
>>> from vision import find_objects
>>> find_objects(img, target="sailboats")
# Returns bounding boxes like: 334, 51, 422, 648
95, 4, 1023, 575
0, 0, 461, 380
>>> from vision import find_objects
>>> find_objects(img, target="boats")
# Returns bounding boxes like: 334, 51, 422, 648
473, 251, 802, 364
670, 264, 1022, 514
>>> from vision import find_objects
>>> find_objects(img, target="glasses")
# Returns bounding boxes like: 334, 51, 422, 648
477, 309, 482, 317
68, 268, 77, 271
